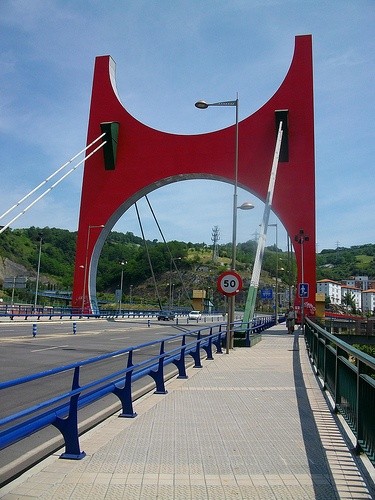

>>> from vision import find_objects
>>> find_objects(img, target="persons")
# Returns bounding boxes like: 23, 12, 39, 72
287, 307, 296, 334
285, 309, 289, 327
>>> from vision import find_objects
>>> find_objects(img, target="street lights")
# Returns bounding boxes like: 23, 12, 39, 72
294, 228, 309, 327
195, 92, 254, 349
80, 224, 104, 315
118, 258, 128, 313
170, 257, 182, 301
34, 232, 46, 311
258, 223, 284, 325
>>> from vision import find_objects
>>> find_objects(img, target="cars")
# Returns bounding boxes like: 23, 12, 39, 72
188, 310, 202, 320
158, 311, 176, 321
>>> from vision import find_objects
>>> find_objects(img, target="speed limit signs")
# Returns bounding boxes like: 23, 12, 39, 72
218, 271, 242, 297
